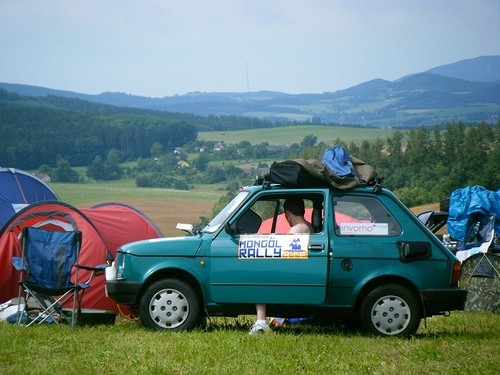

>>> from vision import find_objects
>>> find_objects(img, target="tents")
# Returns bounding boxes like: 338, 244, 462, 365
0, 166, 60, 234
0, 200, 166, 320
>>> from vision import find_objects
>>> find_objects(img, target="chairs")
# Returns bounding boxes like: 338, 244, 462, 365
11, 228, 108, 328
311, 201, 322, 233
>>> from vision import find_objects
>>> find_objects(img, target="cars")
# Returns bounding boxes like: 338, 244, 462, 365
105, 183, 467, 339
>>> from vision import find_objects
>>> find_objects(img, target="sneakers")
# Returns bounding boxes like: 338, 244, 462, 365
245, 324, 271, 335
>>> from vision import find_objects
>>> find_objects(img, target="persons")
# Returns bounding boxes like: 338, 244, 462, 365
248, 197, 315, 338
